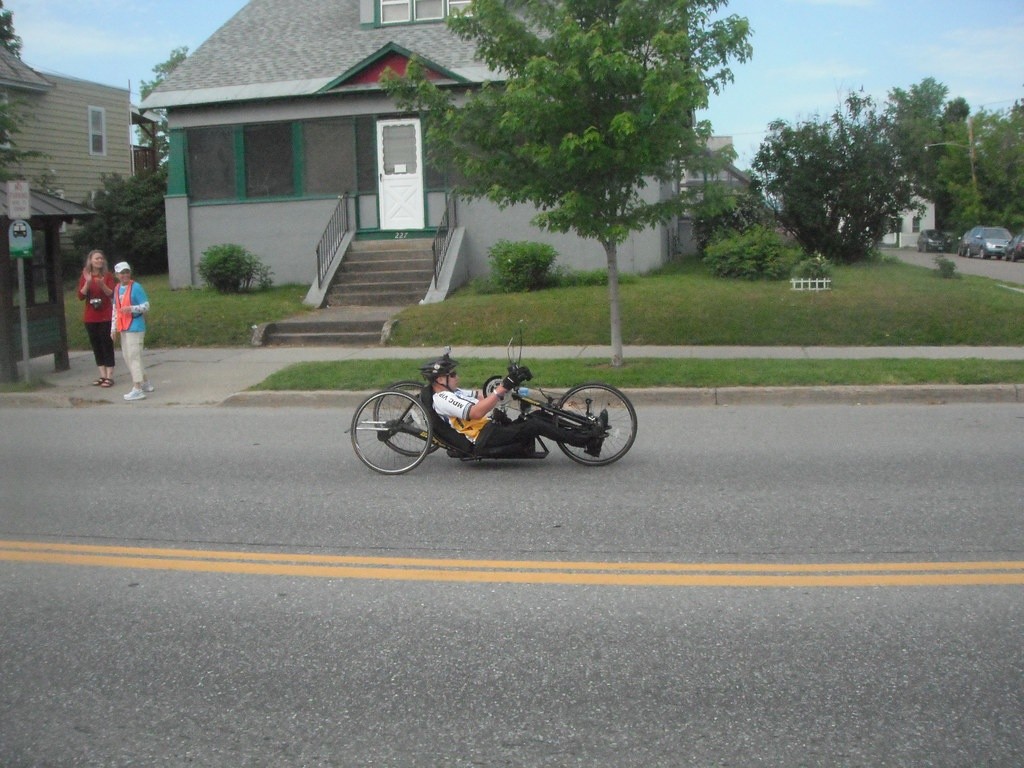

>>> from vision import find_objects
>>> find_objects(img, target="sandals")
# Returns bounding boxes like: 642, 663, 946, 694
100, 377, 114, 387
92, 377, 105, 386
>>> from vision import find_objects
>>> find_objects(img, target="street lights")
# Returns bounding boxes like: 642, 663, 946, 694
924, 116, 980, 225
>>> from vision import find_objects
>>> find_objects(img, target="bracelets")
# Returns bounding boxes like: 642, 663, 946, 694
493, 389, 503, 400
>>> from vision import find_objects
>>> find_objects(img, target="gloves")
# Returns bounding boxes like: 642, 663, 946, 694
500, 366, 533, 391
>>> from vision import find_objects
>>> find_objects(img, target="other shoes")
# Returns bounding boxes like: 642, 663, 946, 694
133, 383, 154, 391
123, 387, 146, 401
583, 409, 609, 458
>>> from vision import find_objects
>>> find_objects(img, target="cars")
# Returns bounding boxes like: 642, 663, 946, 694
958, 227, 1013, 259
1005, 234, 1024, 262
917, 229, 953, 253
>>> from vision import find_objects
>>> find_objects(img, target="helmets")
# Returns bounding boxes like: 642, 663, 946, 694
417, 359, 459, 383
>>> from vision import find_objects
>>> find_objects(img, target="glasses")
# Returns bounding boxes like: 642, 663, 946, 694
448, 370, 457, 379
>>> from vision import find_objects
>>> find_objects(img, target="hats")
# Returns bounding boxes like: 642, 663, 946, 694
114, 261, 131, 273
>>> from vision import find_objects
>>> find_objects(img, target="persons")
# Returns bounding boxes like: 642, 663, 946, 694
78, 249, 115, 387
420, 356, 607, 450
109, 262, 154, 400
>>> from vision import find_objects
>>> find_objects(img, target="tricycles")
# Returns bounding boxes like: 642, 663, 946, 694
343, 325, 639, 476
